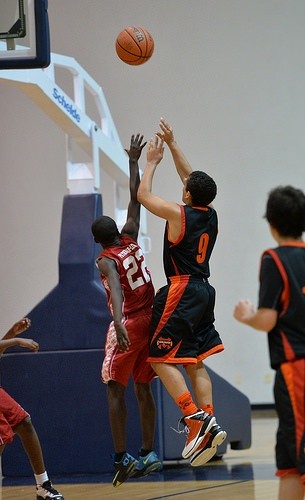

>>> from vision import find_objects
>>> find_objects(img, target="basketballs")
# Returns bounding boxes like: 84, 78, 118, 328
114, 24, 155, 65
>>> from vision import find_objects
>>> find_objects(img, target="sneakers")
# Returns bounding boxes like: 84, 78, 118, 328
129, 451, 163, 480
189, 421, 227, 467
35, 479, 64, 500
112, 452, 145, 488
170, 408, 216, 459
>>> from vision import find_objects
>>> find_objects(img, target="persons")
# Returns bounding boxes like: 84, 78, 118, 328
91, 133, 160, 488
232, 185, 305, 500
137, 116, 227, 466
0, 318, 65, 500
